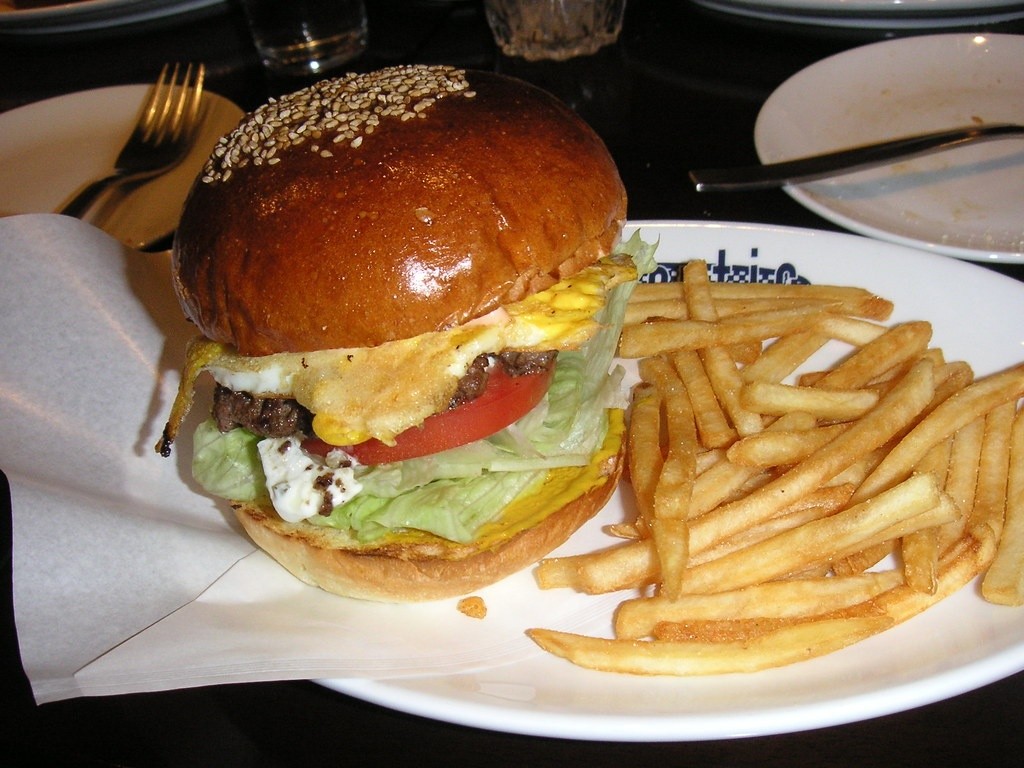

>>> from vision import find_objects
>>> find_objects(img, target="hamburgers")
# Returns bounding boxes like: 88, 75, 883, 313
157, 67, 657, 607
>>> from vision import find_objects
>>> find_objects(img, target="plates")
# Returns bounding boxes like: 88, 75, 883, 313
312, 219, 1024, 742
0, 84, 247, 251
0, 0, 229, 42
0, 0, 143, 22
755, 32, 1024, 264
730, 0, 1024, 18
692, 0, 1024, 29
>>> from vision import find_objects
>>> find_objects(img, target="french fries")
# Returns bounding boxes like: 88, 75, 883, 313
525, 263, 1024, 679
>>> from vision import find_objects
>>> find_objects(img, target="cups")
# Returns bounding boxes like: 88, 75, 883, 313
244, 0, 370, 76
484, 0, 626, 62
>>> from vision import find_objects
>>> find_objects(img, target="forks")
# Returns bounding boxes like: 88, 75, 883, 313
61, 61, 205, 220
